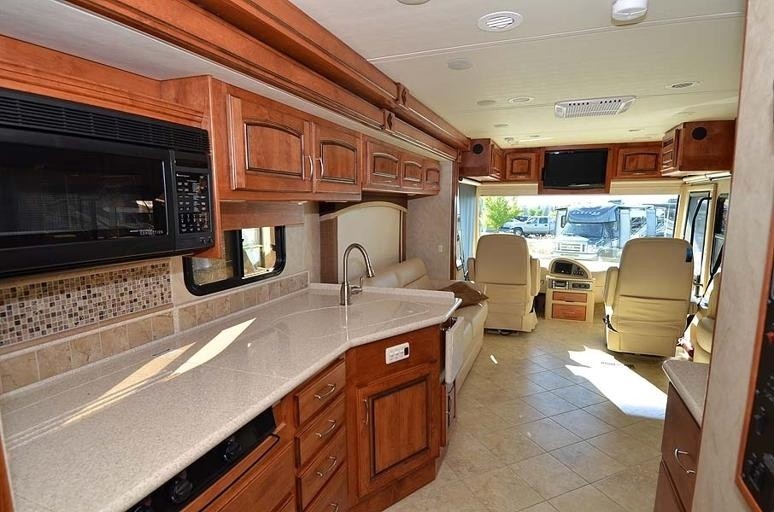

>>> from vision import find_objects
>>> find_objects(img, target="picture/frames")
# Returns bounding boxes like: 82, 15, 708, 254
735, 194, 774, 512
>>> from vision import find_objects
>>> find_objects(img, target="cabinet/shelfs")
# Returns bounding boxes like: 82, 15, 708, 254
653, 383, 702, 512
127, 312, 465, 512
211, 78, 734, 202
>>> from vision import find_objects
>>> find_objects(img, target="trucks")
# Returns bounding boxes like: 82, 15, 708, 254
552, 201, 668, 261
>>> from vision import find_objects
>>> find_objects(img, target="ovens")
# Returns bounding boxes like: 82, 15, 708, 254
0, 86, 217, 280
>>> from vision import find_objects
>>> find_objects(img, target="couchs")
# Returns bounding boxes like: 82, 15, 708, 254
349, 257, 489, 396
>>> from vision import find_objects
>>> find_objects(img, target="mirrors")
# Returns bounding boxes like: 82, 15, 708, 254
183, 226, 286, 296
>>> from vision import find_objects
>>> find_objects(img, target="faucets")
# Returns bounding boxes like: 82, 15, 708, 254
339, 241, 374, 306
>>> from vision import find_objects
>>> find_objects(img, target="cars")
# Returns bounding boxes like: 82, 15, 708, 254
500, 214, 549, 237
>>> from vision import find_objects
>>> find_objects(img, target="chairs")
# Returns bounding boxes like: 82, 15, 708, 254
467, 234, 722, 365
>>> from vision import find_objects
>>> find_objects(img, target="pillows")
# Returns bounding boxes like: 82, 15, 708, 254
438, 282, 488, 310
